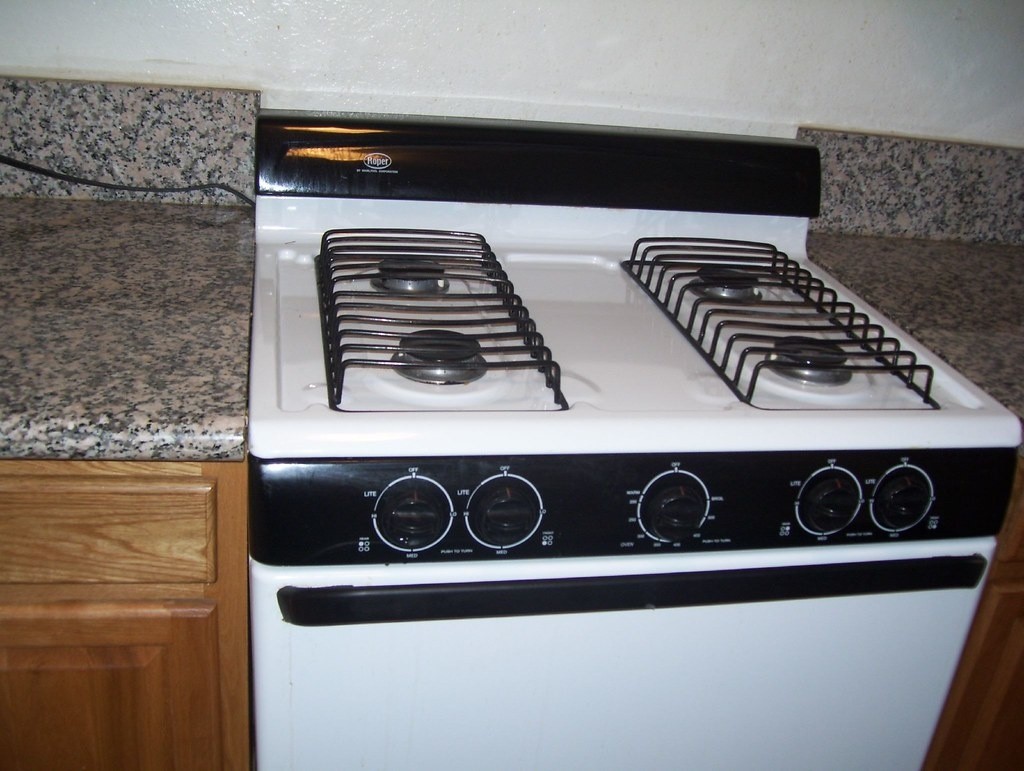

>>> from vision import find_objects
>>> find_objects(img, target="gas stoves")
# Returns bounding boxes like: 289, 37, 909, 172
243, 104, 1024, 562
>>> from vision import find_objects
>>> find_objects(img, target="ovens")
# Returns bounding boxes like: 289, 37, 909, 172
246, 539, 994, 771
